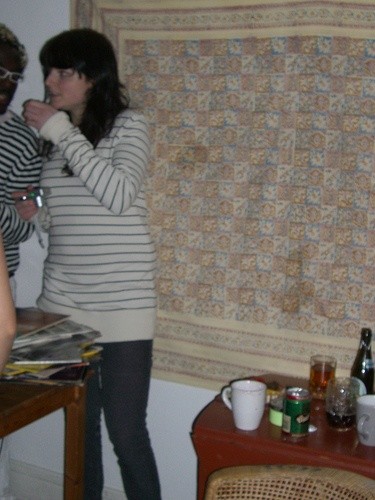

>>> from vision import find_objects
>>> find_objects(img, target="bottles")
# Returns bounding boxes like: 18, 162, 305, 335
350, 328, 374, 395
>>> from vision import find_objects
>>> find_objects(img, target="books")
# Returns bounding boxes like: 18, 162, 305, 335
4, 307, 105, 387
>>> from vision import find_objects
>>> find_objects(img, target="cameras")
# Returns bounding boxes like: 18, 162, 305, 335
21, 186, 52, 208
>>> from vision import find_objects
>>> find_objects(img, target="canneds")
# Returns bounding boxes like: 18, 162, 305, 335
281, 387, 311, 439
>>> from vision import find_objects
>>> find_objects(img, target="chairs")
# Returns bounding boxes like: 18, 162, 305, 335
205, 464, 375, 500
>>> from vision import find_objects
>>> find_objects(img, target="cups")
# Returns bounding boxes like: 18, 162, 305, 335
356, 394, 375, 447
221, 380, 267, 432
308, 355, 337, 400
326, 377, 361, 432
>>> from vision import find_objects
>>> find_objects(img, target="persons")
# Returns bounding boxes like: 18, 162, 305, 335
0, 21, 44, 378
11, 27, 162, 500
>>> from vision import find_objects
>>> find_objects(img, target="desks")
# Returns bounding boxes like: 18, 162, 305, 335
0, 367, 96, 500
189, 374, 375, 500
